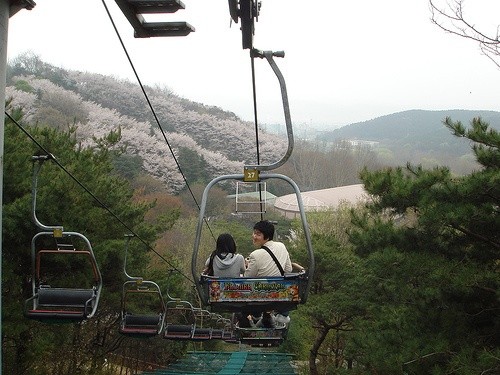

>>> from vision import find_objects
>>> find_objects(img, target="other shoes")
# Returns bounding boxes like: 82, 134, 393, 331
275, 314, 290, 329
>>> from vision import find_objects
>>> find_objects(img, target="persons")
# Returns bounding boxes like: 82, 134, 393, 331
205, 233, 245, 278
245, 220, 293, 277
238, 312, 290, 329
